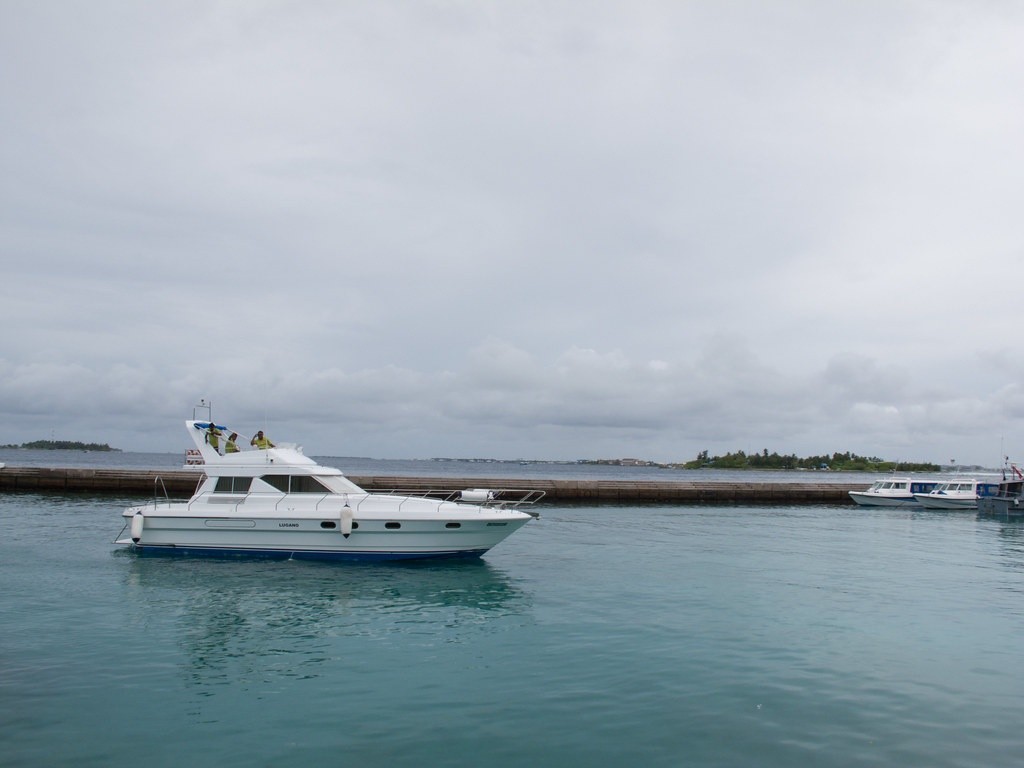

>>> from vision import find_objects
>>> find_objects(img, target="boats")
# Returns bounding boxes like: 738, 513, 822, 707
848, 460, 939, 507
111, 397, 546, 562
977, 436, 1024, 515
913, 479, 1000, 509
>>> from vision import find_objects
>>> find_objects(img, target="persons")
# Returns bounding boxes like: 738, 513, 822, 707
205, 423, 222, 453
225, 433, 240, 454
250, 431, 276, 450
